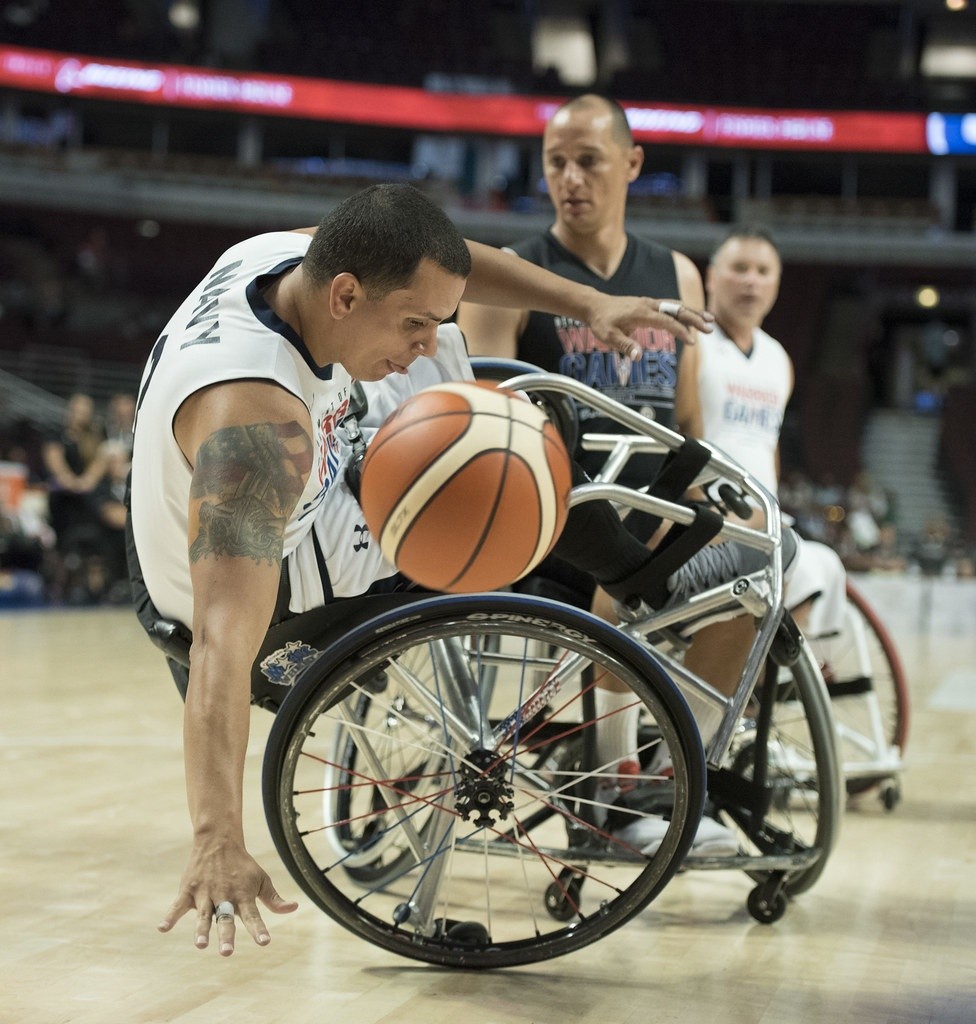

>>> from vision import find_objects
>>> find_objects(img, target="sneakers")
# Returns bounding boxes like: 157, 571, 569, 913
677, 815, 738, 875
611, 523, 801, 646
590, 773, 693, 867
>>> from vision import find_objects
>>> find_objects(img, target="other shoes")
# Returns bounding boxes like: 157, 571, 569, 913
720, 716, 805, 780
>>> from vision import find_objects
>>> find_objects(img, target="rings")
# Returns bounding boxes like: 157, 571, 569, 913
215, 901, 235, 919
659, 302, 680, 317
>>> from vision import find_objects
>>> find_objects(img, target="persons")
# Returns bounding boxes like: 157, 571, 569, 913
696, 224, 843, 766
454, 92, 780, 858
125, 180, 798, 956
1, 387, 974, 605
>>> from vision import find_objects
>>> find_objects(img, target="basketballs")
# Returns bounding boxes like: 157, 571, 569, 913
360, 383, 572, 594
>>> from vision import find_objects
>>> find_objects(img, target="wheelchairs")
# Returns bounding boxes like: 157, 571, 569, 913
262, 358, 838, 972
732, 576, 911, 814
